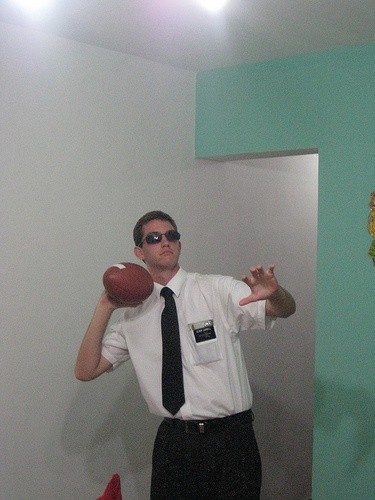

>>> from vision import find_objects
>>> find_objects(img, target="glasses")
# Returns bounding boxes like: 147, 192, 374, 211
138, 230, 180, 248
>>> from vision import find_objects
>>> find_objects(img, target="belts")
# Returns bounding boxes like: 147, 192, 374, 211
164, 410, 253, 436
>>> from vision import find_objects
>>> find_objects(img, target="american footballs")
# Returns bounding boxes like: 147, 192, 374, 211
103, 262, 155, 305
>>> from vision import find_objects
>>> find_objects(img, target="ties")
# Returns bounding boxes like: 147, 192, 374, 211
161, 287, 185, 415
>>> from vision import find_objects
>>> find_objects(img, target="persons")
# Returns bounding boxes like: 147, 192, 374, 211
75, 210, 296, 500
